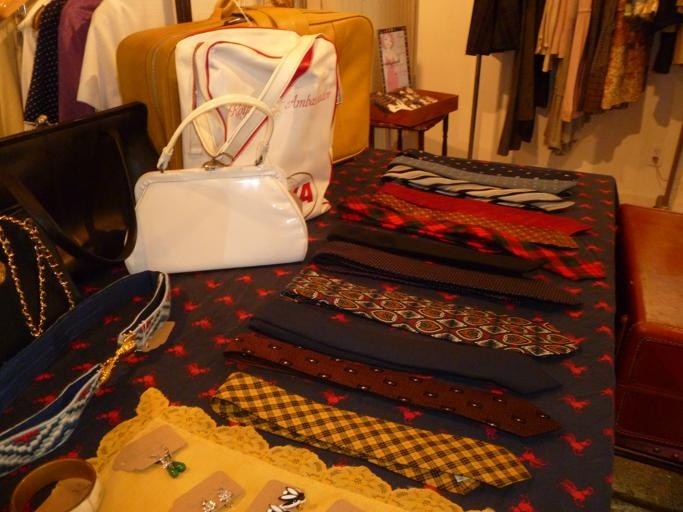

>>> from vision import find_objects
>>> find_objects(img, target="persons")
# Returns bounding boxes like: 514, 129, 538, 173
382, 32, 400, 92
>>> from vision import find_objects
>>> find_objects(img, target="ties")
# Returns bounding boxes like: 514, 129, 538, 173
211, 153, 607, 496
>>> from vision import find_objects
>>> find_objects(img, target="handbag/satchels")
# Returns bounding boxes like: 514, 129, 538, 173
0, 101, 157, 362
116, 1, 376, 274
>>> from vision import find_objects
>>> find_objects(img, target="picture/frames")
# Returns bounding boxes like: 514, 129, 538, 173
377, 25, 413, 96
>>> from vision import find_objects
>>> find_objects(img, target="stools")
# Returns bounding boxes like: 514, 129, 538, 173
369, 113, 449, 154
